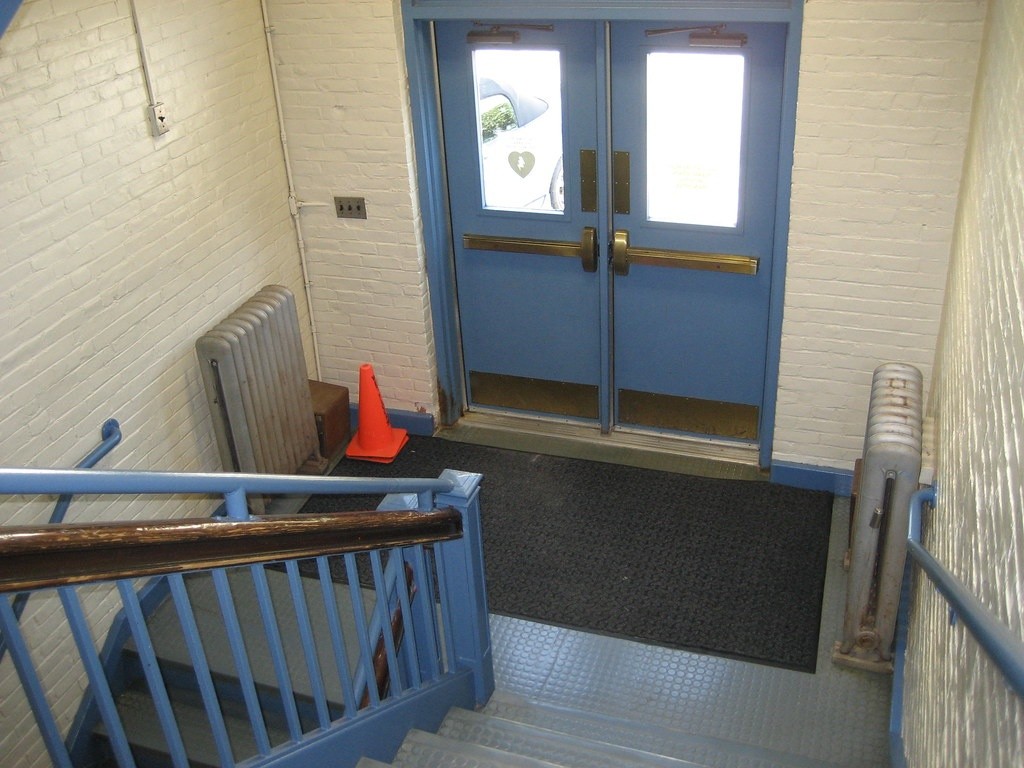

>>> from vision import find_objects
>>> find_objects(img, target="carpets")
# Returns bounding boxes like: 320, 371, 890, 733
262, 433, 834, 676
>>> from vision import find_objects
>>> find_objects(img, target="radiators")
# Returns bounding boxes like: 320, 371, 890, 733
193, 285, 332, 520
832, 361, 926, 671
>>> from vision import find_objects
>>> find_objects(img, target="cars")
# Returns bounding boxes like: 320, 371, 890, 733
472, 50, 564, 214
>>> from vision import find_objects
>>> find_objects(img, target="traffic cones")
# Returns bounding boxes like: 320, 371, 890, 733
345, 362, 410, 464
